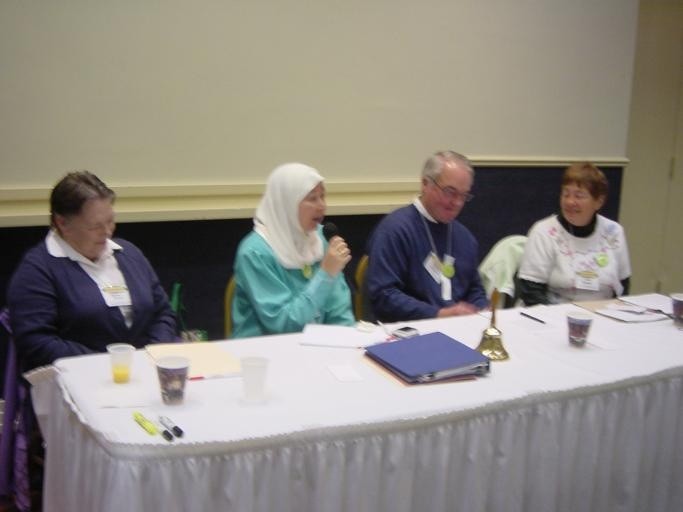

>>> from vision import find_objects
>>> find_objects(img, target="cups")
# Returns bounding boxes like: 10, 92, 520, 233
107, 344, 136, 383
568, 312, 593, 347
671, 293, 683, 318
156, 358, 188, 405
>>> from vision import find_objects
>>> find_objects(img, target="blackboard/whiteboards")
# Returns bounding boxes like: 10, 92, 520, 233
0, 0, 637, 200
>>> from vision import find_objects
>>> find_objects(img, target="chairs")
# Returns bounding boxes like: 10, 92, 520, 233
354, 256, 376, 323
489, 238, 540, 311
226, 278, 353, 351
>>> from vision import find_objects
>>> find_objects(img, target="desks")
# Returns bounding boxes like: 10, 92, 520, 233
50, 293, 683, 512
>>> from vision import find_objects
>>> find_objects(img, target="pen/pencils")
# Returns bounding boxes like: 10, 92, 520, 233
520, 312, 546, 324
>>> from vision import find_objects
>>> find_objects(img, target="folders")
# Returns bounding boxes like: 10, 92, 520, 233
365, 332, 490, 384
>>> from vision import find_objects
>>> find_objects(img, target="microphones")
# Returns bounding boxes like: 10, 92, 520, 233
322, 222, 360, 293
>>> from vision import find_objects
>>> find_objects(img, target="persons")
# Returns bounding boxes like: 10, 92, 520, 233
8, 170, 177, 428
233, 161, 354, 339
517, 161, 632, 306
365, 150, 489, 322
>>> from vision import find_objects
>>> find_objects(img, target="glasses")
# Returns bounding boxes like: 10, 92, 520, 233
425, 174, 475, 202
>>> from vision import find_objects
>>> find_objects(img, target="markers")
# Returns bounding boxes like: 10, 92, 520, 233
149, 417, 173, 440
158, 414, 183, 437
132, 411, 158, 435
